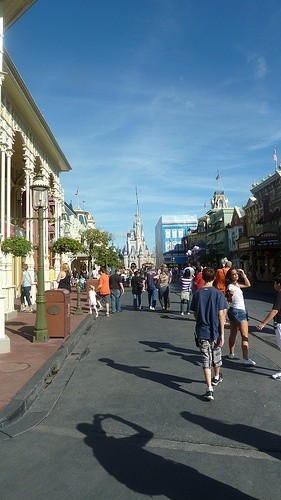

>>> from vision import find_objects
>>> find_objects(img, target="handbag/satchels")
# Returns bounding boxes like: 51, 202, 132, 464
155, 276, 161, 289
180, 291, 189, 300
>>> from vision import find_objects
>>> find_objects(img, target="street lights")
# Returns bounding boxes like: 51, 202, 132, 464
28, 171, 51, 343
249, 236, 257, 292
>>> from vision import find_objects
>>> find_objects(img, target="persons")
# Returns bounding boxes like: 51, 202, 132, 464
56, 262, 72, 294
49, 264, 56, 290
255, 273, 281, 380
188, 267, 225, 401
178, 268, 192, 316
17, 262, 34, 313
71, 262, 176, 320
193, 265, 209, 292
33, 267, 40, 282
214, 257, 235, 325
224, 266, 257, 366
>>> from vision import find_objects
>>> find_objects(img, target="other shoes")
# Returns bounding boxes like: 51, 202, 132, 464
272, 371, 281, 380
229, 353, 234, 358
243, 359, 256, 366
187, 313, 191, 315
202, 388, 214, 400
179, 312, 184, 315
212, 373, 223, 385
149, 306, 155, 310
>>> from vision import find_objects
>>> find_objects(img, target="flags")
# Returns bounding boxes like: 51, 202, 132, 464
216, 174, 221, 181
